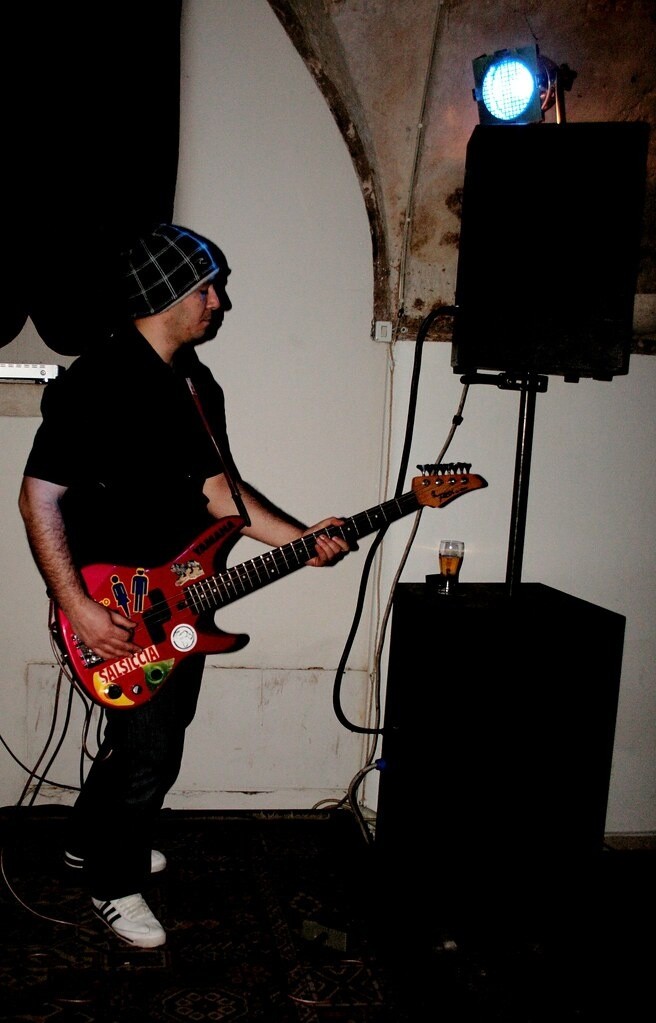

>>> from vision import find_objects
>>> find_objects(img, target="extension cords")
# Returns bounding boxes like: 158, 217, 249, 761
303, 918, 352, 952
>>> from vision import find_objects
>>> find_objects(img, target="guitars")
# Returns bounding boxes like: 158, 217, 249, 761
56, 461, 489, 711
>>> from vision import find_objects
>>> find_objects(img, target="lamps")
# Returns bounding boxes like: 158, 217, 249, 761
471, 44, 557, 126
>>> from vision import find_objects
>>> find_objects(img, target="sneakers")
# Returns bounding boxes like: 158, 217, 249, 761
91, 892, 166, 948
65, 847, 167, 873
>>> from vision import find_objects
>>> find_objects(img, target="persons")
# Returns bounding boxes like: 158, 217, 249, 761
18, 222, 351, 948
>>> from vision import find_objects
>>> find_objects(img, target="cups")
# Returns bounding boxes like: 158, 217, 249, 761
437, 540, 465, 596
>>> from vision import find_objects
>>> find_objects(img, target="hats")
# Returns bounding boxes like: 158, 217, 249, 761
122, 223, 219, 322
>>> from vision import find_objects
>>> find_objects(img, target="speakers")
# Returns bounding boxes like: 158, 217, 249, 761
450, 121, 650, 382
